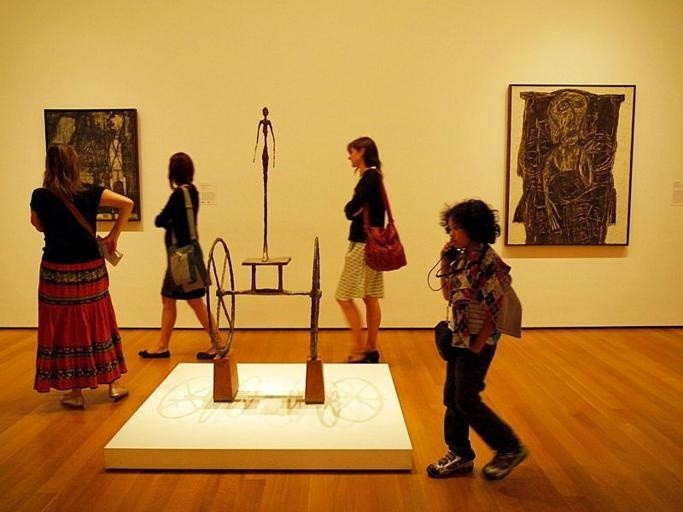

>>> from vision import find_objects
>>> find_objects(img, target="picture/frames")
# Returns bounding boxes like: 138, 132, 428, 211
501, 82, 637, 248
44, 107, 143, 235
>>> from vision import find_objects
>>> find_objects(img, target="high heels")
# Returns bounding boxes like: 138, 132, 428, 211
345, 349, 380, 364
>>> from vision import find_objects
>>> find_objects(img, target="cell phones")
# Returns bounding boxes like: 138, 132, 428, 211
445, 246, 461, 261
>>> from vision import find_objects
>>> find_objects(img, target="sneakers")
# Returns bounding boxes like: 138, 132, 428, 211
427, 452, 474, 479
482, 441, 529, 481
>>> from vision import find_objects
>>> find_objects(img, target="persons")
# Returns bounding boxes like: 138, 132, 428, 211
424, 198, 531, 482
139, 152, 226, 359
335, 137, 387, 364
29, 142, 134, 409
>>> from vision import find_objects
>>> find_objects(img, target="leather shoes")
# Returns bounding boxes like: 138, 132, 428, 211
138, 348, 170, 358
196, 346, 226, 359
61, 395, 85, 408
109, 385, 128, 399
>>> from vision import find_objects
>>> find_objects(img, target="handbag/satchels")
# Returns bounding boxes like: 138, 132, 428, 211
362, 219, 409, 272
167, 243, 196, 286
434, 320, 456, 362
